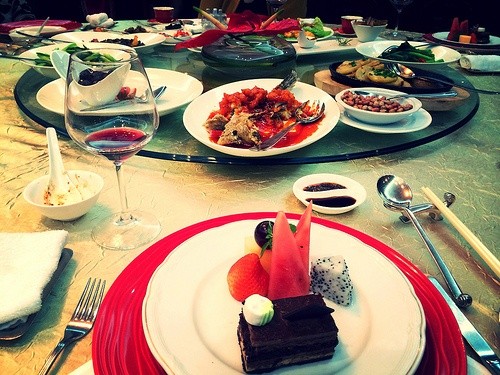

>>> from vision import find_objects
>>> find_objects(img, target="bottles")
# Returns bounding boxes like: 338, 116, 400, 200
201, 8, 225, 34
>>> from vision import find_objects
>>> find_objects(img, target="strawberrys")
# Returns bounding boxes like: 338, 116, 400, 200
226, 253, 269, 302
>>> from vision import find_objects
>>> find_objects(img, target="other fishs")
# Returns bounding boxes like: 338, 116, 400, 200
205, 70, 326, 149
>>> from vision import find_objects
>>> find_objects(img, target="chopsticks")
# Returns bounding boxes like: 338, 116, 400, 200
420, 185, 500, 284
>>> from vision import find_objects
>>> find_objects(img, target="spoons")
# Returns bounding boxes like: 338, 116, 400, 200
249, 100, 325, 151
353, 91, 458, 101
93, 86, 166, 111
386, 63, 500, 96
40, 127, 82, 205
377, 174, 473, 310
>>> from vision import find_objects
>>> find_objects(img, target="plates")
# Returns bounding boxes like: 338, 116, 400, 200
293, 173, 367, 215
8, 19, 500, 158
91, 212, 467, 375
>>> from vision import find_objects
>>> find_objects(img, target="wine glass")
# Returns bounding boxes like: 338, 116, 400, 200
385, 0, 409, 41
64, 48, 161, 250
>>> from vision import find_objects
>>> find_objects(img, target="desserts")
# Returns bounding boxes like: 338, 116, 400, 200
237, 293, 339, 373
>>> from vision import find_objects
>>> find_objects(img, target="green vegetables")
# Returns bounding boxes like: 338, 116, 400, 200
36, 44, 119, 67
378, 41, 445, 63
350, 56, 398, 78
302, 17, 332, 37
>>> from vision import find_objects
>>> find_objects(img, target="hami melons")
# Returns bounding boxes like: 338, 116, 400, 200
459, 35, 471, 43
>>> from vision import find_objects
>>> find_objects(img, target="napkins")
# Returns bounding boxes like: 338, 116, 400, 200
0, 228, 69, 321
459, 53, 500, 71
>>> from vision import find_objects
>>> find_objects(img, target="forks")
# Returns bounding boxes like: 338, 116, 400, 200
36, 279, 112, 375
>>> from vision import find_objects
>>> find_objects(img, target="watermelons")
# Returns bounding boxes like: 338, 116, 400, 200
265, 201, 312, 298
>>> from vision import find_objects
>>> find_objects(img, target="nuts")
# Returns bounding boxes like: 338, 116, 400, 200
341, 91, 412, 113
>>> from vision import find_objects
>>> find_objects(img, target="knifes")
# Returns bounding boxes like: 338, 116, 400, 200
426, 276, 500, 375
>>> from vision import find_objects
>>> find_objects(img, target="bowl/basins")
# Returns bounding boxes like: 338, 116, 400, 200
10, 26, 65, 44
350, 19, 385, 42
335, 87, 422, 125
22, 171, 104, 222
18, 43, 136, 78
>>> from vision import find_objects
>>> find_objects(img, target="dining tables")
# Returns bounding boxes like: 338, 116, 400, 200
0, 20, 500, 375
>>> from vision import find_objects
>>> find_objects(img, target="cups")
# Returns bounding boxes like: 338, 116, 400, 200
341, 15, 364, 34
154, 7, 174, 23
